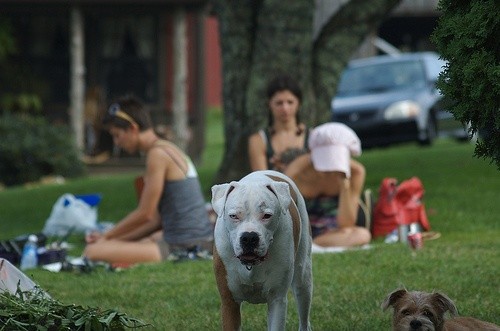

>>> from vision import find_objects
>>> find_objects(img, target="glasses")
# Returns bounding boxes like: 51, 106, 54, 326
108, 103, 137, 128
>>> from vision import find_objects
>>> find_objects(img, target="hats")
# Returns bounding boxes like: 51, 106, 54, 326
308, 122, 361, 179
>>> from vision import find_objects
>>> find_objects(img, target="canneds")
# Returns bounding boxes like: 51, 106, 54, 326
407, 230, 424, 249
20, 241, 39, 271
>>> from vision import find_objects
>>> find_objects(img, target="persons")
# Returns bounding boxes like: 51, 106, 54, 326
249, 76, 372, 251
78, 97, 210, 265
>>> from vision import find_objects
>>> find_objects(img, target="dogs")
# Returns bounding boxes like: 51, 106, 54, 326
382, 289, 500, 331
211, 170, 313, 331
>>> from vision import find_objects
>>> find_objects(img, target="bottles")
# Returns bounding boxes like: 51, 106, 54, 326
20, 234, 38, 270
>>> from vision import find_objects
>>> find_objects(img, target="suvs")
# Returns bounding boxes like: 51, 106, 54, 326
328, 49, 475, 148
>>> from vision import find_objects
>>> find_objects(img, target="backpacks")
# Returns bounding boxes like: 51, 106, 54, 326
373, 176, 430, 240
